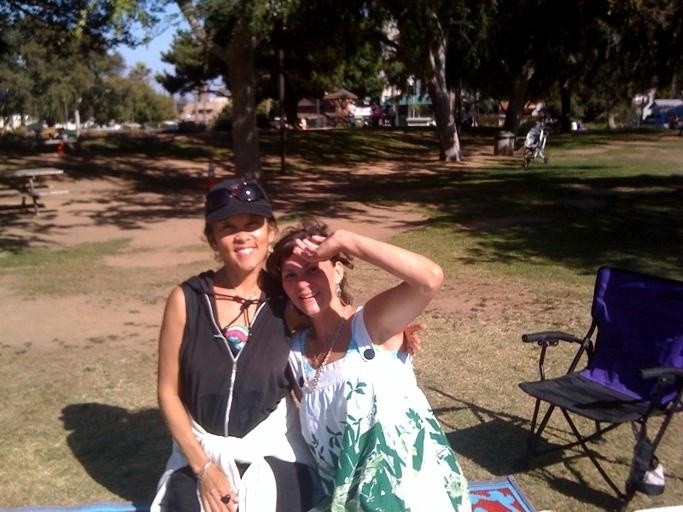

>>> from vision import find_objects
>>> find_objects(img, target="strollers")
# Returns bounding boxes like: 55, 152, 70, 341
520, 122, 550, 169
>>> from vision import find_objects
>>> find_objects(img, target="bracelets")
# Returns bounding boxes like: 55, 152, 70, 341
194, 462, 210, 479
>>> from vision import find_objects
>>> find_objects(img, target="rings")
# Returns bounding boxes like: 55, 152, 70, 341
220, 494, 230, 504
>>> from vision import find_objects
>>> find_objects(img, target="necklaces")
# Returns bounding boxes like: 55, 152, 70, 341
302, 301, 353, 394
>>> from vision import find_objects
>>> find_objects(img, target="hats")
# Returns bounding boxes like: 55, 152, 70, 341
204, 177, 272, 224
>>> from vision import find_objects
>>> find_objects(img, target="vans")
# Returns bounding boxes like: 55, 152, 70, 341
405, 116, 437, 127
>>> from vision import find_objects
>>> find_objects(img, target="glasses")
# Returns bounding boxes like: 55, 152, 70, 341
206, 185, 263, 212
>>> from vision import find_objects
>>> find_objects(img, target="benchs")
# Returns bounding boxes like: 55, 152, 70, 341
0, 166, 70, 219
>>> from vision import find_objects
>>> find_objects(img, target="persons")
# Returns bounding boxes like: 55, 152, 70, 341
148, 176, 426, 512
263, 222, 475, 511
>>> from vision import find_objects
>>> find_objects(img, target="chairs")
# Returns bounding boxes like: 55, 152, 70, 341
517, 264, 683, 506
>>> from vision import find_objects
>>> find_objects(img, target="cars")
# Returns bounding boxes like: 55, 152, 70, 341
157, 120, 178, 129
